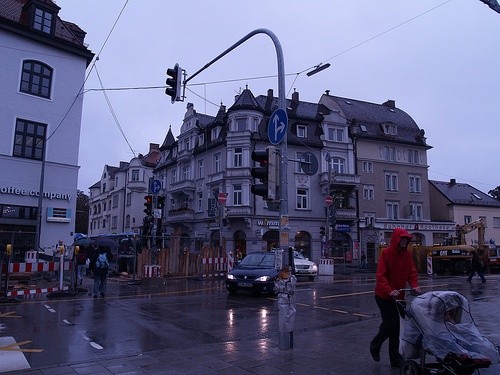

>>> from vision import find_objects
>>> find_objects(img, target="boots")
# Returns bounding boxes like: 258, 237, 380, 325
370, 336, 381, 362
388, 352, 405, 367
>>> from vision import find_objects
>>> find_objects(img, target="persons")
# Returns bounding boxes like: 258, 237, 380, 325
88, 246, 113, 298
465, 250, 488, 284
71, 232, 95, 284
442, 311, 455, 328
368, 227, 422, 373
358, 251, 366, 270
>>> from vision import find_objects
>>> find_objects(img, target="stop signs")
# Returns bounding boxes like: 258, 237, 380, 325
218, 193, 226, 204
325, 196, 333, 205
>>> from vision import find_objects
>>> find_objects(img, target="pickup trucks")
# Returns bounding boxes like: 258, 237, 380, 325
487, 248, 500, 268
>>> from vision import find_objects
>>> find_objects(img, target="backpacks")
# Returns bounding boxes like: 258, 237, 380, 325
95, 249, 110, 270
74, 246, 87, 265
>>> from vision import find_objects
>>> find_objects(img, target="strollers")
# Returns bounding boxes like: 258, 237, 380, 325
392, 289, 500, 375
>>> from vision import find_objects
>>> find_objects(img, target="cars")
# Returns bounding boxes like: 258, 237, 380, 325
294, 251, 318, 280
226, 251, 277, 292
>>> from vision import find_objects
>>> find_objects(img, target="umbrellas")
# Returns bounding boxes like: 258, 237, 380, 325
86, 235, 120, 249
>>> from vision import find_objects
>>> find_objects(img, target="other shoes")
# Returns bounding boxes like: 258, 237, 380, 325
100, 292, 104, 297
79, 279, 83, 285
93, 295, 97, 298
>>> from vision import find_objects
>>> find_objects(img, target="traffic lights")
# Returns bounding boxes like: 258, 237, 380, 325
157, 196, 166, 209
144, 195, 152, 215
320, 226, 325, 239
251, 147, 269, 200
166, 65, 177, 102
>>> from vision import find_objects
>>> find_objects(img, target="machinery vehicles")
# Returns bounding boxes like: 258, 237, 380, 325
377, 218, 491, 273
